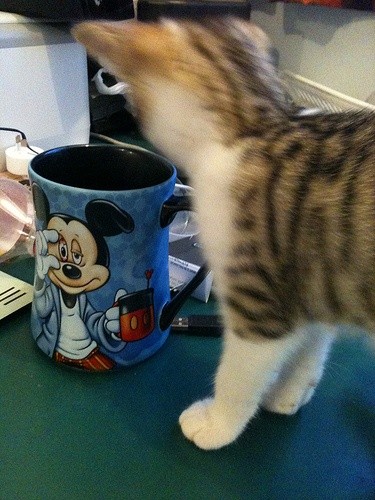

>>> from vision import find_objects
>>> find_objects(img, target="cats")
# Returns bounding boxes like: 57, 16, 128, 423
70, 15, 375, 451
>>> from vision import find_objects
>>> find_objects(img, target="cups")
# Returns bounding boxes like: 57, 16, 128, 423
29, 146, 211, 373
